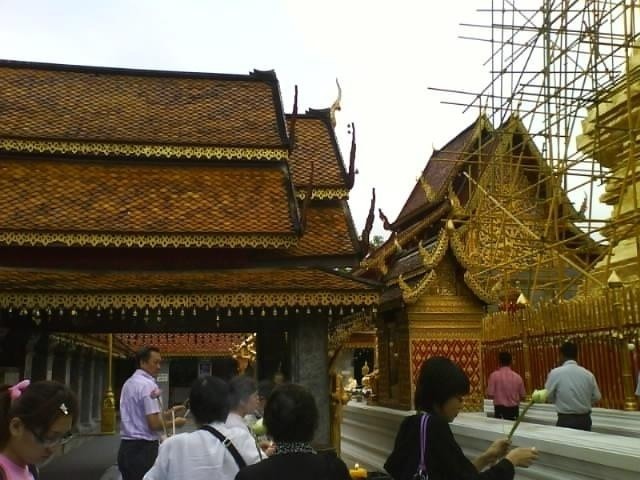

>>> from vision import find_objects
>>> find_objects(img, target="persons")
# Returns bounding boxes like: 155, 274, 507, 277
542, 342, 603, 433
484, 350, 526, 422
382, 353, 538, 480
116, 343, 188, 479
231, 382, 351, 479
0, 377, 76, 480
140, 375, 269, 479
224, 376, 259, 431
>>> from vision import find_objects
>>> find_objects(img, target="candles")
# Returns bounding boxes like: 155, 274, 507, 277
349, 461, 368, 479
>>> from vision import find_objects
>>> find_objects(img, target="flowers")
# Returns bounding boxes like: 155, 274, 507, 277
505, 388, 551, 445
251, 414, 269, 465
150, 387, 170, 440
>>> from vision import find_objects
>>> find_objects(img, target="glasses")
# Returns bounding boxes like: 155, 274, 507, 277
24, 421, 74, 448
